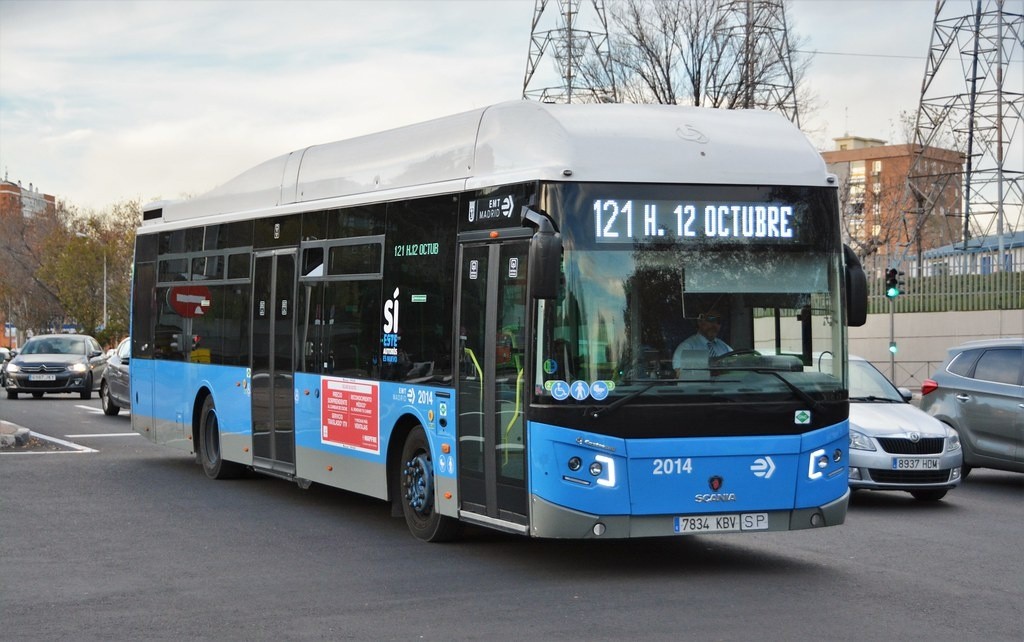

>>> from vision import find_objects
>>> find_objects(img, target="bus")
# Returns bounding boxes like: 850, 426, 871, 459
128, 99, 869, 545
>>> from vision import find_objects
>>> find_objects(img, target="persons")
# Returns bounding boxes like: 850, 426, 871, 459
673, 303, 738, 386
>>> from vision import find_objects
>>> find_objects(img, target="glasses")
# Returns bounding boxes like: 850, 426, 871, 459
706, 315, 724, 325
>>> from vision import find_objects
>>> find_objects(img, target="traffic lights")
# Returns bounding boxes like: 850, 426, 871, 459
888, 341, 897, 356
191, 334, 200, 351
897, 271, 906, 296
885, 268, 899, 299
170, 333, 184, 353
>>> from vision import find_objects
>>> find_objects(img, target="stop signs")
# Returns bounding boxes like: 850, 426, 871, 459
165, 287, 212, 319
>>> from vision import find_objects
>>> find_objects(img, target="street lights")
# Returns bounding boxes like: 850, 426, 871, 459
9, 290, 32, 351
75, 231, 108, 332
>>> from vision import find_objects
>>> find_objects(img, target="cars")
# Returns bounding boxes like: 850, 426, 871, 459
6, 332, 116, 400
0, 346, 21, 387
755, 349, 962, 502
101, 336, 131, 417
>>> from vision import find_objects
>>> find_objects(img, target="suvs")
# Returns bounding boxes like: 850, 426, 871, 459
917, 337, 1024, 480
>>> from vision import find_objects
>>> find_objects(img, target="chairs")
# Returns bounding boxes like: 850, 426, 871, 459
36, 342, 53, 353
326, 339, 367, 375
660, 309, 730, 368
153, 324, 183, 347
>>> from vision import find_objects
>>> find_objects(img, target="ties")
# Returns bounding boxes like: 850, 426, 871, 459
707, 342, 718, 358
